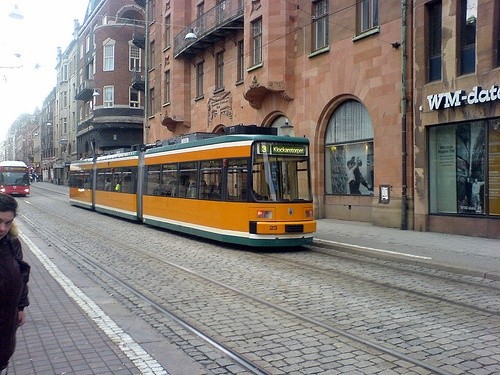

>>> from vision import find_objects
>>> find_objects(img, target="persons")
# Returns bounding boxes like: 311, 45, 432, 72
347, 156, 371, 194
0, 193, 31, 372
472, 179, 485, 211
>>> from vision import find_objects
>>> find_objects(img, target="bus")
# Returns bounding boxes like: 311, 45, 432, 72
0, 160, 30, 197
69, 125, 316, 247
